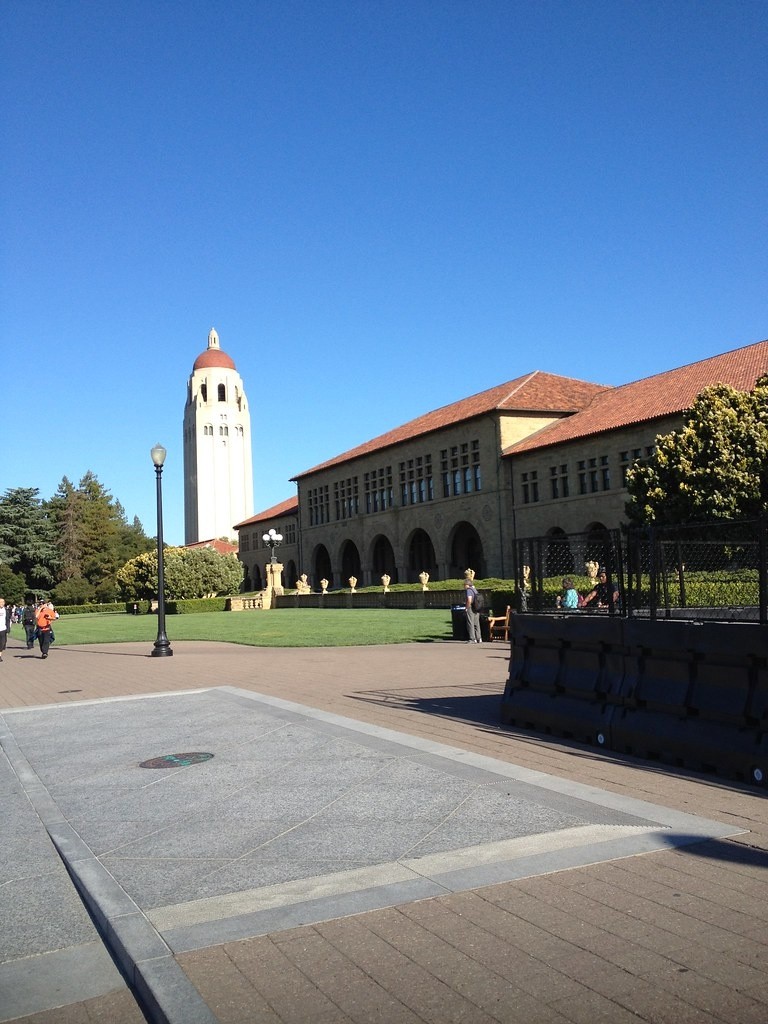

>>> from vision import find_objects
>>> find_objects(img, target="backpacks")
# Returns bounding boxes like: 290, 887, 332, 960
24, 609, 34, 625
468, 588, 483, 612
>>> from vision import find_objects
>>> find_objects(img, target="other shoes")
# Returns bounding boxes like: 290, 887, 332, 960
27, 644, 34, 650
466, 639, 482, 645
41, 653, 47, 659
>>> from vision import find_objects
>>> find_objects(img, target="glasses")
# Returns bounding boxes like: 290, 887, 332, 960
39, 604, 43, 605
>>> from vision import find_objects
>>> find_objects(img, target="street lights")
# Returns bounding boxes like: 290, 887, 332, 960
151, 443, 173, 657
262, 529, 283, 563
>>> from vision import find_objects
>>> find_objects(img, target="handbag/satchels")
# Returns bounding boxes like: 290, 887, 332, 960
578, 594, 587, 607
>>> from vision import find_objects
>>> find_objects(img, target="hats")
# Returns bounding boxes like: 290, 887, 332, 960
39, 600, 45, 603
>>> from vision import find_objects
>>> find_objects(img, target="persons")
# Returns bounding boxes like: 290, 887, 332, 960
578, 567, 620, 611
0, 596, 61, 661
463, 578, 482, 644
556, 578, 585, 611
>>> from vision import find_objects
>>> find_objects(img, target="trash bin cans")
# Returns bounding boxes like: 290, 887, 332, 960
450, 603, 471, 641
